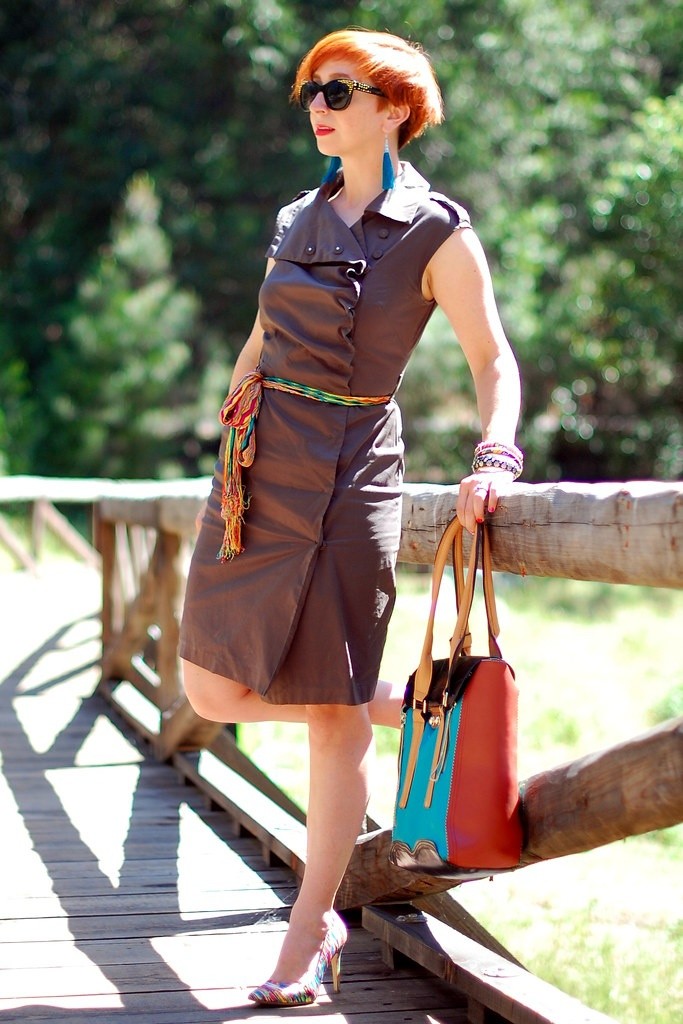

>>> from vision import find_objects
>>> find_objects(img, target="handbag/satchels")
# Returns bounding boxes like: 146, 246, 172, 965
388, 516, 518, 878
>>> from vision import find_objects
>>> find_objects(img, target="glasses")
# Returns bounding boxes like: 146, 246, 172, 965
299, 82, 388, 110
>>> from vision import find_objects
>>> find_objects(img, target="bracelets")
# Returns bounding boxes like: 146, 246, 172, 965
473, 440, 524, 461
473, 447, 523, 471
471, 454, 522, 478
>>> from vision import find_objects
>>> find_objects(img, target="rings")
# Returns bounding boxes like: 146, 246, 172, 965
474, 486, 488, 494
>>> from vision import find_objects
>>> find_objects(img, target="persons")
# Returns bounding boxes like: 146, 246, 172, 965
177, 26, 528, 1008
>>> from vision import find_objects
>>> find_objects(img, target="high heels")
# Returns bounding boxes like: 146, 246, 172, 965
248, 911, 351, 1007
